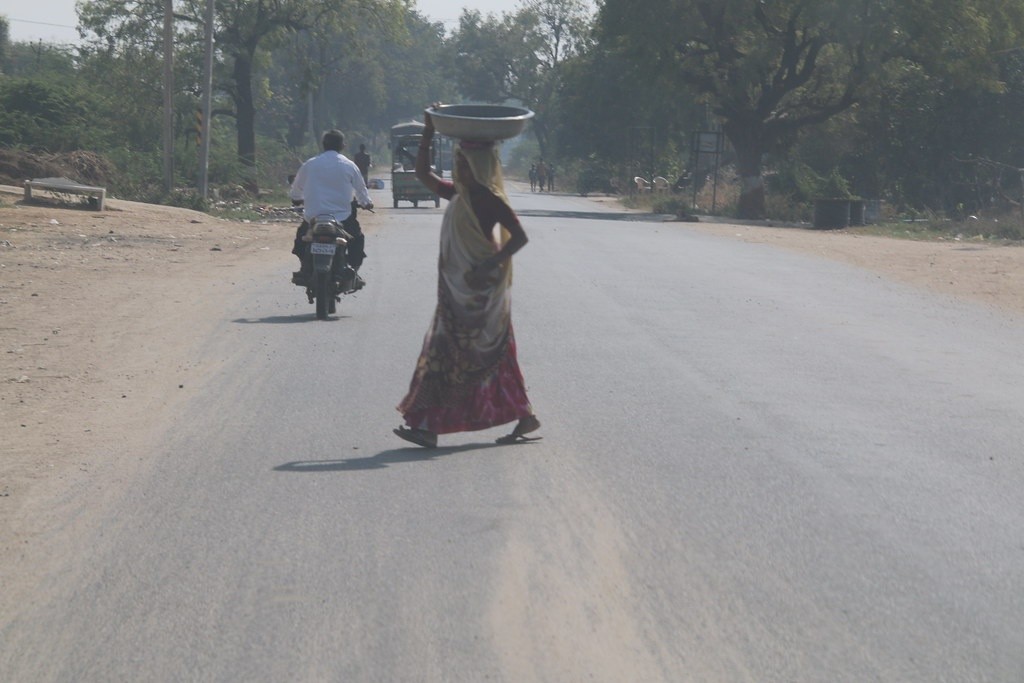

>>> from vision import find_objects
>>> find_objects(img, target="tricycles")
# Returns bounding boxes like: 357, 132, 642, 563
386, 119, 441, 210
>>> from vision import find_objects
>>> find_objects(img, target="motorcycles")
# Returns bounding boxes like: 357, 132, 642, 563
293, 198, 375, 319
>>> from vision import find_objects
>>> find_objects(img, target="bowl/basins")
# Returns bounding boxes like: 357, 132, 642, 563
426, 105, 536, 140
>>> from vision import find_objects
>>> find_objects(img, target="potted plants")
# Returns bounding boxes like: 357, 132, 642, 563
814, 171, 853, 230
850, 195, 869, 226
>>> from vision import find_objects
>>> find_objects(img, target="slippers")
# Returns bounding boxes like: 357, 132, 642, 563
392, 425, 437, 447
496, 433, 542, 444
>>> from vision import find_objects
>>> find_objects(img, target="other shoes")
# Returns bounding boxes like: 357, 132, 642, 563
298, 267, 312, 284
339, 277, 366, 291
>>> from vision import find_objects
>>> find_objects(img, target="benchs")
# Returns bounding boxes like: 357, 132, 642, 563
24, 179, 105, 213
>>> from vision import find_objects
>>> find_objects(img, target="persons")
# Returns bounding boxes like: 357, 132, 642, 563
393, 101, 541, 449
353, 145, 371, 189
290, 130, 372, 290
529, 159, 556, 193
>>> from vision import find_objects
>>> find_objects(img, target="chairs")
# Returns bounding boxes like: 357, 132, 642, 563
633, 177, 651, 194
654, 177, 671, 196
